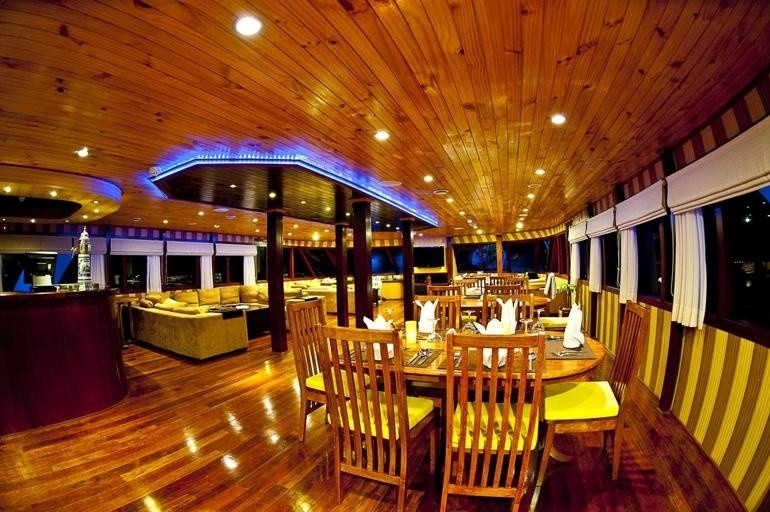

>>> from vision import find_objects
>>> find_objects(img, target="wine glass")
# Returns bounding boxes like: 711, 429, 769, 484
519, 308, 547, 336
427, 310, 477, 348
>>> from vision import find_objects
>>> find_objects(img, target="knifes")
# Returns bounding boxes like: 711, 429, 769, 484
418, 351, 434, 365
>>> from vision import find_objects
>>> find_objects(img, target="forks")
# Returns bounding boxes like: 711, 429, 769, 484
550, 349, 588, 358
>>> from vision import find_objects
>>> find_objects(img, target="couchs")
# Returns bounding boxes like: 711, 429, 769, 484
129, 275, 327, 360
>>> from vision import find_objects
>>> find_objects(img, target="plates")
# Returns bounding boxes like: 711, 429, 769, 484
235, 305, 249, 310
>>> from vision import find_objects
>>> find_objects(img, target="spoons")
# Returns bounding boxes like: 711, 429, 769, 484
528, 351, 536, 371
412, 347, 428, 366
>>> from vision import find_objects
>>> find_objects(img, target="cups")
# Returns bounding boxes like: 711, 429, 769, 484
405, 320, 418, 344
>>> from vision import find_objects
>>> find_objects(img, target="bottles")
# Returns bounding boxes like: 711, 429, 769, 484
76, 225, 92, 286
